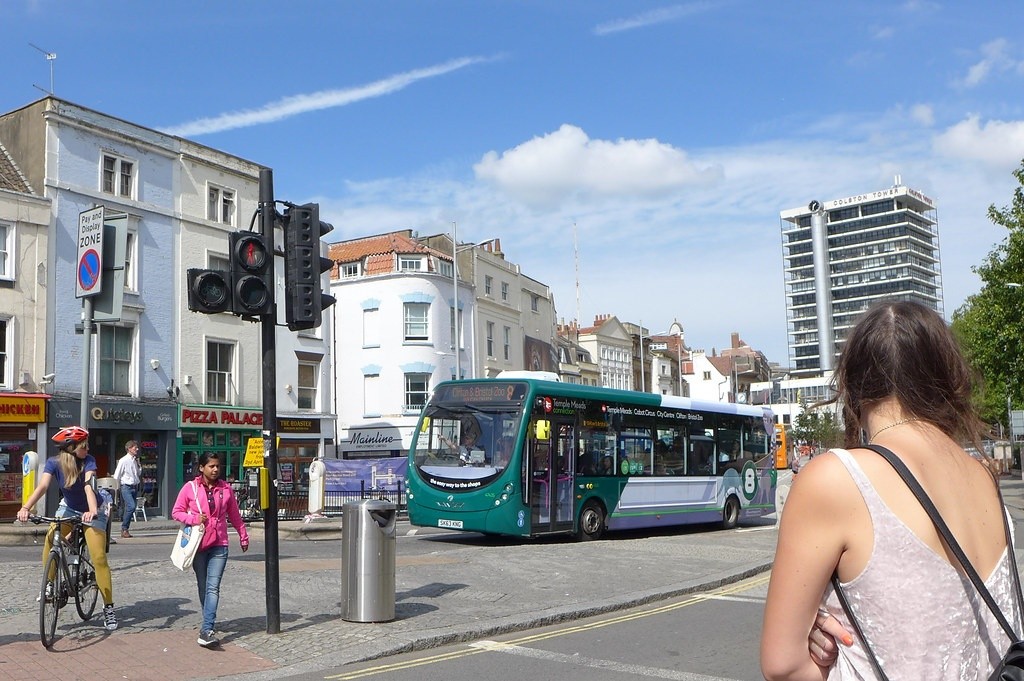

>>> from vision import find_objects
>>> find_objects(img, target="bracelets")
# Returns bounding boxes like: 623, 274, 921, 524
23, 507, 30, 511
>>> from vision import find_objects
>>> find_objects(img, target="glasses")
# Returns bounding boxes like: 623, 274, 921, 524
78, 442, 90, 449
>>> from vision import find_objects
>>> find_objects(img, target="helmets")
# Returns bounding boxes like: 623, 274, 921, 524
51, 426, 89, 443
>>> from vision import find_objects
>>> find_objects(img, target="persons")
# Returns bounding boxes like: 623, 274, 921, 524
172, 451, 248, 646
227, 474, 240, 496
792, 457, 800, 476
760, 299, 1024, 681
114, 440, 142, 538
438, 432, 481, 466
577, 439, 740, 477
17, 426, 118, 631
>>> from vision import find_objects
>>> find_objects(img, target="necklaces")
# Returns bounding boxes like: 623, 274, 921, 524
868, 418, 923, 445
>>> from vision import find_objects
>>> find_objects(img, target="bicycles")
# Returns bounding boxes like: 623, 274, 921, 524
15, 512, 99, 647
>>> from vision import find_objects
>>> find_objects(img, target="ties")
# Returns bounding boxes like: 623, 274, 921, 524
465, 447, 472, 461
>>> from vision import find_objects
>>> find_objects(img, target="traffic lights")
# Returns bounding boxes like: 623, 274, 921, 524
283, 203, 336, 331
187, 230, 275, 315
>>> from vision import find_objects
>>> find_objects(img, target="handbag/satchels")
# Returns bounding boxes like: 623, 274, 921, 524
169, 510, 206, 572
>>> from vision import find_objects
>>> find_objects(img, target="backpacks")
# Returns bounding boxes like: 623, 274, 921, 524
832, 446, 1024, 681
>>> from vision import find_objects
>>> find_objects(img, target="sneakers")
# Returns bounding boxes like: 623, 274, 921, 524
198, 630, 219, 647
37, 582, 54, 601
102, 602, 118, 630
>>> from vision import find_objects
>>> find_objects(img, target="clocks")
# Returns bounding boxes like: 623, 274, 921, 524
809, 200, 821, 212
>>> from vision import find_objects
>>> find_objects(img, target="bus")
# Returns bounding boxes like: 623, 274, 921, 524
776, 424, 794, 469
404, 370, 777, 541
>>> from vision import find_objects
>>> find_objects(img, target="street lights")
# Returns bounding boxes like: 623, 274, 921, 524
453, 239, 494, 380
640, 331, 667, 393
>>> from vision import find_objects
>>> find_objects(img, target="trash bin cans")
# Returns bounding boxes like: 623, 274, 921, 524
342, 499, 397, 622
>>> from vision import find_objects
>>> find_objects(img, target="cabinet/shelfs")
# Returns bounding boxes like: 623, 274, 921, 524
138, 446, 158, 490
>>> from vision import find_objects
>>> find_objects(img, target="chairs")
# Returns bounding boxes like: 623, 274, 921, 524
133, 497, 148, 524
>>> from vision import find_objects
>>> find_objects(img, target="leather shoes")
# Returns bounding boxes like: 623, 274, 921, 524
121, 529, 133, 537
